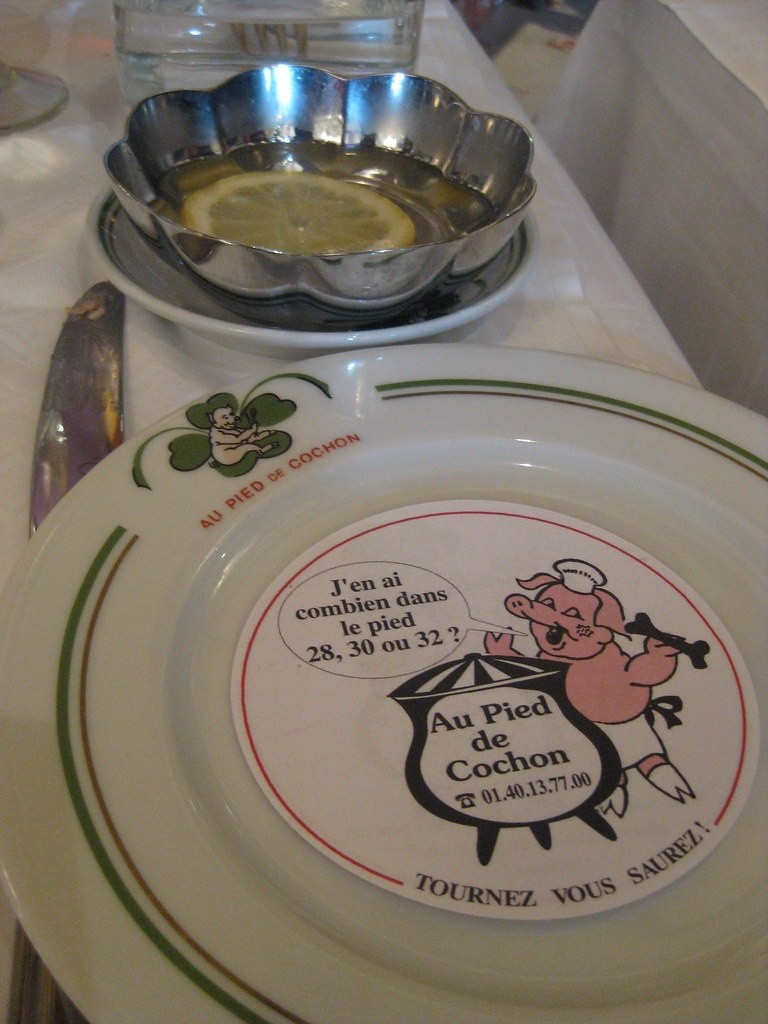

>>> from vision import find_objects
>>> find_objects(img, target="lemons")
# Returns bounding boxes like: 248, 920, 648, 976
181, 170, 417, 253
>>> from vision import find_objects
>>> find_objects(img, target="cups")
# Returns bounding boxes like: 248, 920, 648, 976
111, 0, 244, 103
232, 0, 427, 81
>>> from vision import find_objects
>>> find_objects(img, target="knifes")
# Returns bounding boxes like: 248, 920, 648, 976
8, 278, 127, 1024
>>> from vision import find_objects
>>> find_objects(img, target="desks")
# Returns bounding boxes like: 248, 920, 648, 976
1, 0, 768, 595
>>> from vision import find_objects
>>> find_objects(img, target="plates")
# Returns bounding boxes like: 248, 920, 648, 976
0, 343, 768, 1024
103, 62, 538, 307
83, 182, 539, 361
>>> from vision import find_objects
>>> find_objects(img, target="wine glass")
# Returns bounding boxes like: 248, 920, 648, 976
0, 0, 69, 136
